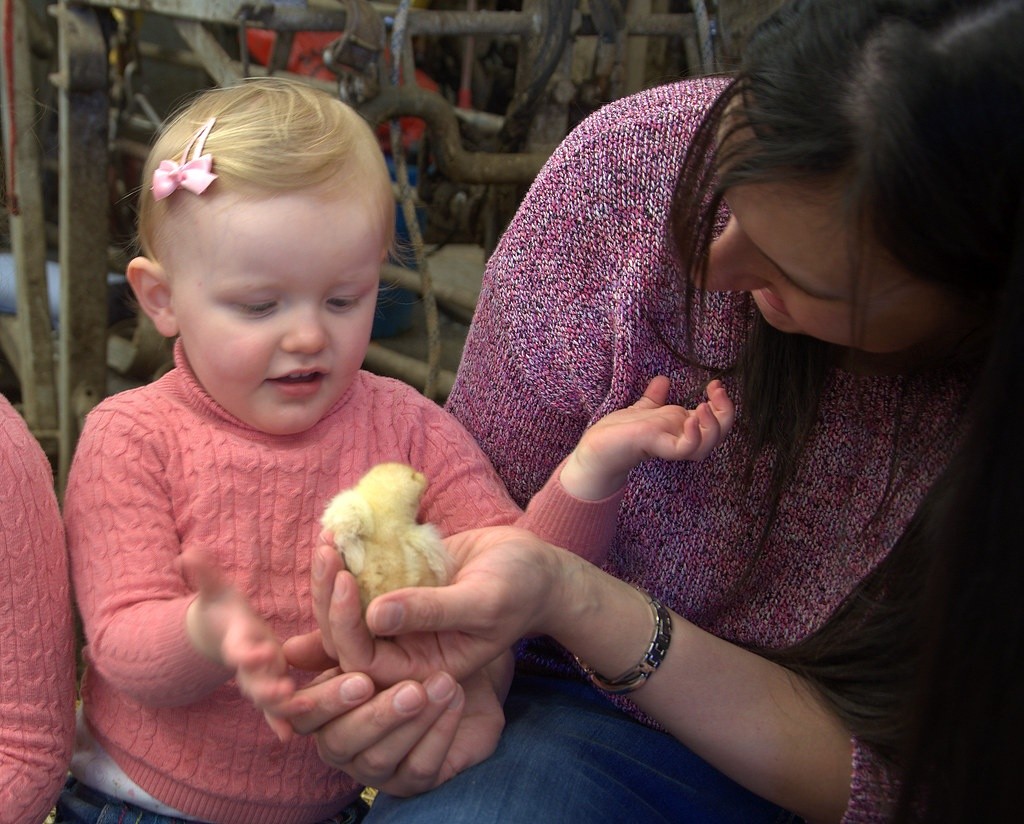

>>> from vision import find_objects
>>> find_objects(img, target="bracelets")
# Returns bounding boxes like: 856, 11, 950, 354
575, 587, 672, 692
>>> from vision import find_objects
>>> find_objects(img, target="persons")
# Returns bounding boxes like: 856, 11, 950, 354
0, 394, 76, 824
54, 80, 737, 824
313, 0, 1024, 824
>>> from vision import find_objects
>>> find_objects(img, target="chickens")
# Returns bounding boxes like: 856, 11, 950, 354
318, 462, 455, 639
